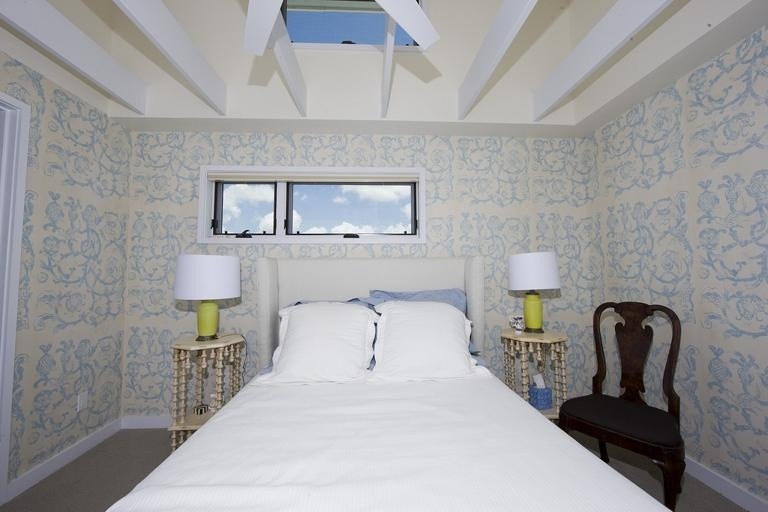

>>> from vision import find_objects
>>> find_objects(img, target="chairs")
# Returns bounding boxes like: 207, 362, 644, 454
558, 298, 686, 510
269, 285, 479, 383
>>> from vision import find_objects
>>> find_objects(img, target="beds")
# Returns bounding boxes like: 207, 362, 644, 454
107, 253, 670, 512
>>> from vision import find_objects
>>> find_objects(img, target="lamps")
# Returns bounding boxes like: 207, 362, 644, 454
507, 249, 561, 334
171, 252, 244, 343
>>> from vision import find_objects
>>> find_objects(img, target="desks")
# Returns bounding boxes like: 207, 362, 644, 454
501, 328, 569, 424
168, 333, 244, 451
